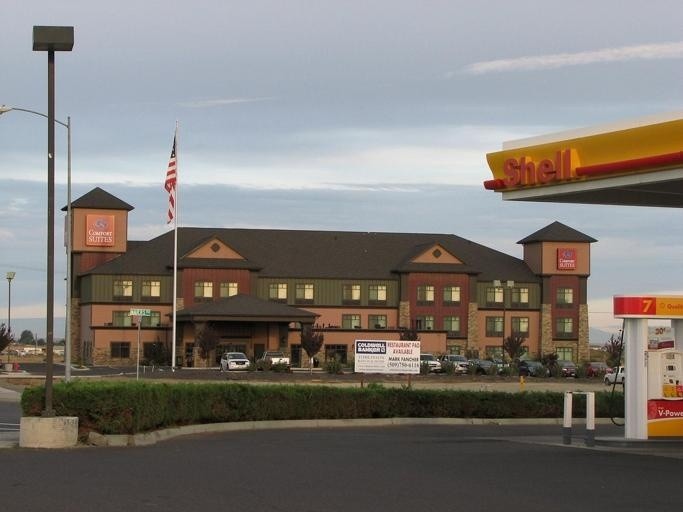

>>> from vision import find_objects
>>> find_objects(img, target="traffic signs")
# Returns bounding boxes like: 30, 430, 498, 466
129, 308, 151, 316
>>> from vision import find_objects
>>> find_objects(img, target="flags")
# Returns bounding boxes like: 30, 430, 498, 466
162, 128, 180, 227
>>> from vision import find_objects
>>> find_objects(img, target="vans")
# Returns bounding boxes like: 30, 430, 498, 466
489, 358, 509, 373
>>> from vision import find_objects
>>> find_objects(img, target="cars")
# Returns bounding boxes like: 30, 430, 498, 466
548, 359, 579, 377
580, 361, 613, 378
219, 352, 249, 370
419, 354, 441, 372
1, 347, 63, 357
438, 354, 472, 374
470, 360, 496, 375
518, 360, 550, 377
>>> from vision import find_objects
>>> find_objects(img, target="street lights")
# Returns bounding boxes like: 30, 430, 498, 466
19, 26, 79, 447
3, 271, 14, 372
0, 105, 72, 387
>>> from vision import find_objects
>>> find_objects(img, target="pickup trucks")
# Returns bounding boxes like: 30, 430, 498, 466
256, 351, 290, 368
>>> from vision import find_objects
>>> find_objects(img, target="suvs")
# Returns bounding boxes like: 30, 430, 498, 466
603, 366, 624, 386
